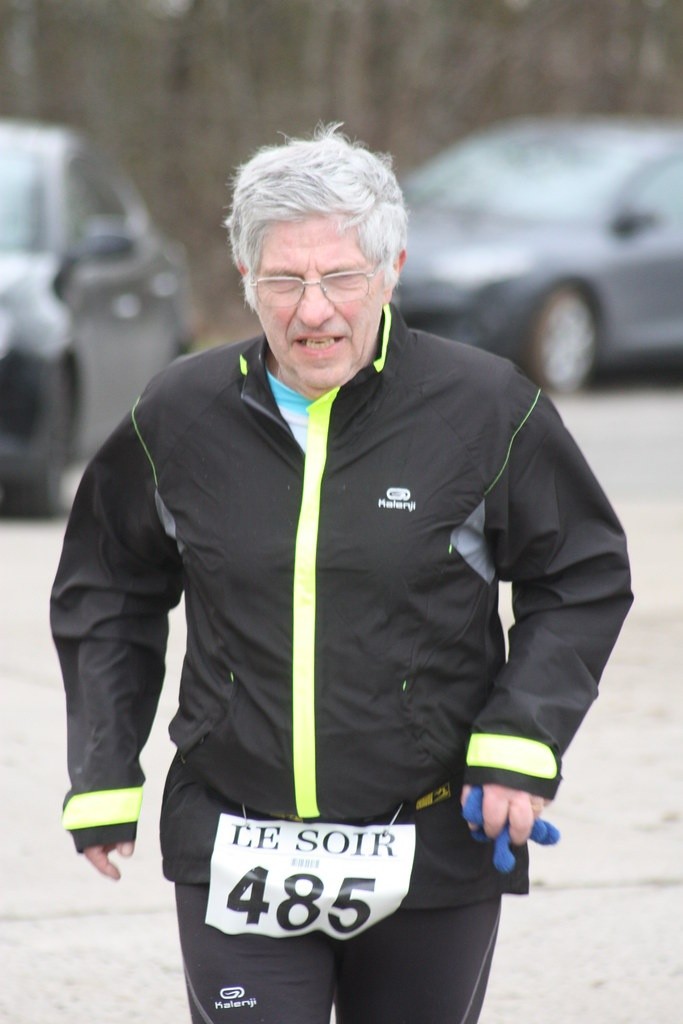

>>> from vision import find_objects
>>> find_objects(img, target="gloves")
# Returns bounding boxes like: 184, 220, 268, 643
465, 788, 560, 871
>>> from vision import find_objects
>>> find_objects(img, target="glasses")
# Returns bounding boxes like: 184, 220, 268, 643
246, 257, 382, 307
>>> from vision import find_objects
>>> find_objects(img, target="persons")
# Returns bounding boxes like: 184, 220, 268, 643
48, 119, 635, 1024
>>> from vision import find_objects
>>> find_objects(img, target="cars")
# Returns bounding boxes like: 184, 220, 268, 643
0, 113, 202, 522
376, 114, 683, 390
